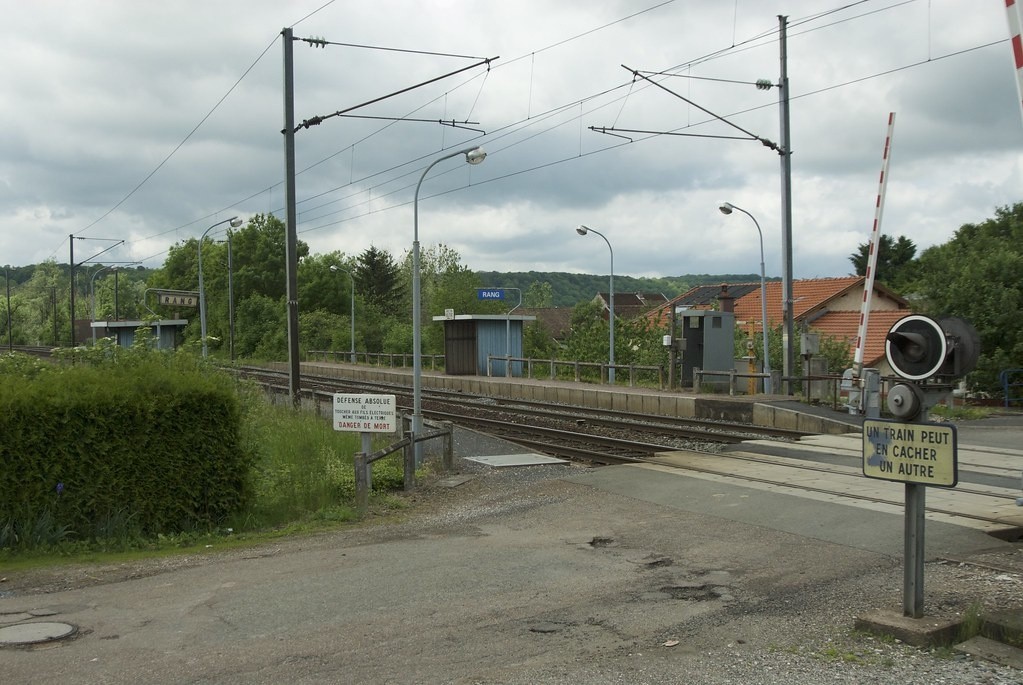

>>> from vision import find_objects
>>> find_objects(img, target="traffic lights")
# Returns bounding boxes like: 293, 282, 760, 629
883, 315, 946, 380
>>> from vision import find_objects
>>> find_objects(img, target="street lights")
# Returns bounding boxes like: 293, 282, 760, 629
719, 201, 771, 393
574, 226, 615, 383
410, 144, 488, 468
91, 263, 117, 345
197, 216, 243, 361
330, 266, 357, 364
